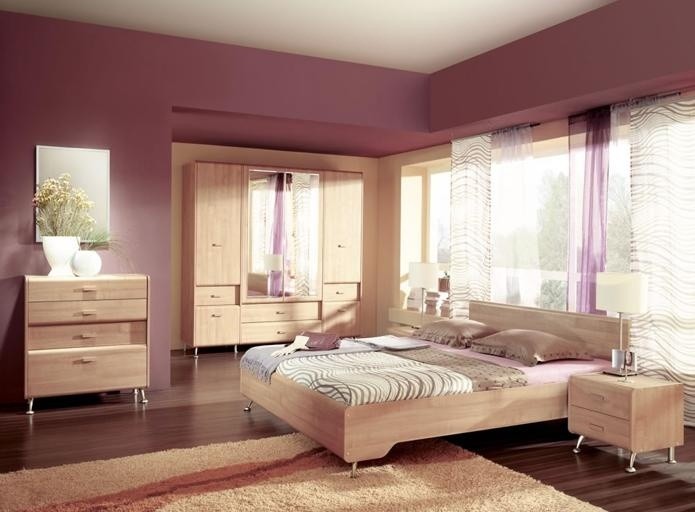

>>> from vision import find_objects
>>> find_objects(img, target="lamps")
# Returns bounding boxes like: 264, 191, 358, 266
408, 262, 438, 317
596, 272, 648, 378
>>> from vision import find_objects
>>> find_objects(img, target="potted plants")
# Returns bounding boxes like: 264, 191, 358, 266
72, 230, 123, 277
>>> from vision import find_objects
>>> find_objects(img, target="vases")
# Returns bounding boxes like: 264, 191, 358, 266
40, 235, 81, 276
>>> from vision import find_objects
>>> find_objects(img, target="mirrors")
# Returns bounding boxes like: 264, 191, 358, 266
249, 168, 322, 300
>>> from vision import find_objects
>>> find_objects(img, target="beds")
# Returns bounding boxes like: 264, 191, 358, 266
240, 299, 631, 479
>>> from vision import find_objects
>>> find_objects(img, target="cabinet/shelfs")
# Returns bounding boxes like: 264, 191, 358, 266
240, 165, 323, 304
23, 274, 151, 415
180, 286, 239, 359
321, 281, 362, 339
321, 170, 364, 283
242, 300, 322, 346
181, 160, 241, 287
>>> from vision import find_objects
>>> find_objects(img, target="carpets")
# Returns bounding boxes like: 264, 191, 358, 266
1, 431, 606, 512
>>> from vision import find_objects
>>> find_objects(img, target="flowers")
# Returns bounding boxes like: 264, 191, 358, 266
31, 173, 96, 236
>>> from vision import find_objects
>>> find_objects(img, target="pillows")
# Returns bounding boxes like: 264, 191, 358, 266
413, 318, 499, 350
470, 328, 594, 367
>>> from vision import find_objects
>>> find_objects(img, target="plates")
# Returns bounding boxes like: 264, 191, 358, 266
603, 368, 639, 377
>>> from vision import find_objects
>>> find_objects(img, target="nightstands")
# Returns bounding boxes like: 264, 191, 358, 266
388, 326, 419, 337
568, 372, 684, 473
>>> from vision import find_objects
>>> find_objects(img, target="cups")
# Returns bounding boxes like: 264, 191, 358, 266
612, 349, 626, 369
